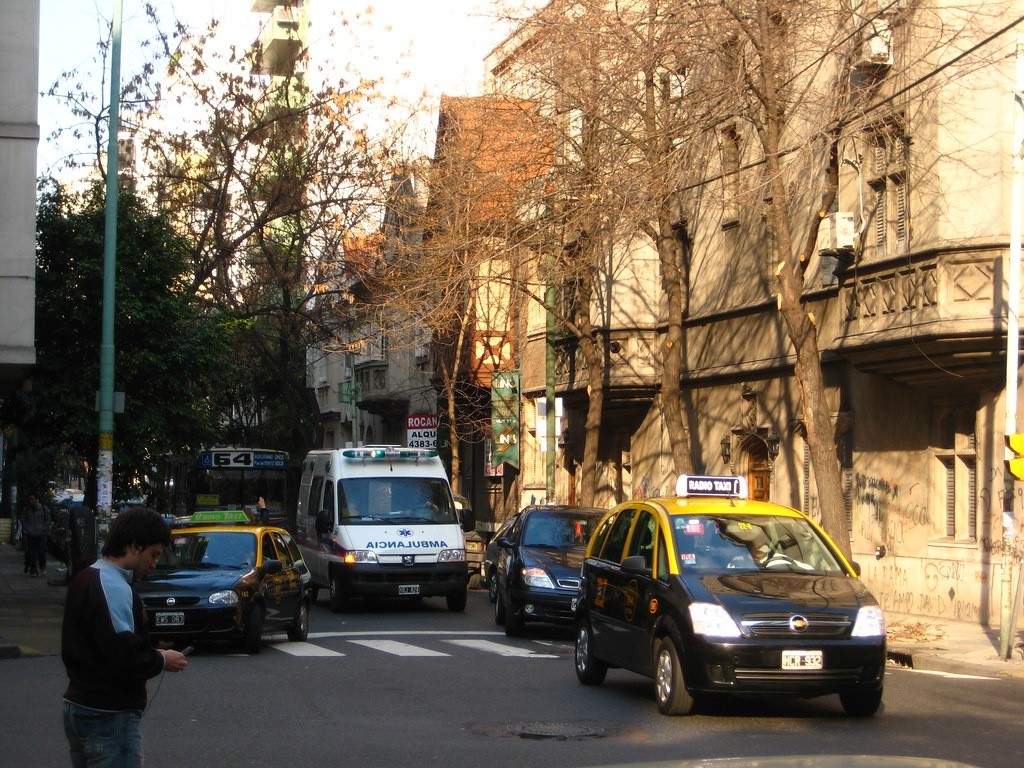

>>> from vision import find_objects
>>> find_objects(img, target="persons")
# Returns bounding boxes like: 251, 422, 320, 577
20, 493, 54, 577
258, 496, 270, 526
59, 505, 188, 768
726, 523, 814, 570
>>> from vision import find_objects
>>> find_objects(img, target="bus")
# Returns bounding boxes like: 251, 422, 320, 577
158, 447, 291, 532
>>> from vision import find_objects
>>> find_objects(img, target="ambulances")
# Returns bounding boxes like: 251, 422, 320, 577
294, 444, 476, 612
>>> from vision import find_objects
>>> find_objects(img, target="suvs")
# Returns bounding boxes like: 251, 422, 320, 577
453, 493, 483, 590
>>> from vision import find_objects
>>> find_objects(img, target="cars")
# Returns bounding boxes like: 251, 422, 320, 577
574, 474, 887, 716
138, 511, 314, 653
50, 480, 85, 502
495, 504, 612, 638
485, 514, 526, 603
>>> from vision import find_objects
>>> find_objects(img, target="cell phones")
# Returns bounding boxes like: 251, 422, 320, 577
182, 645, 194, 655
